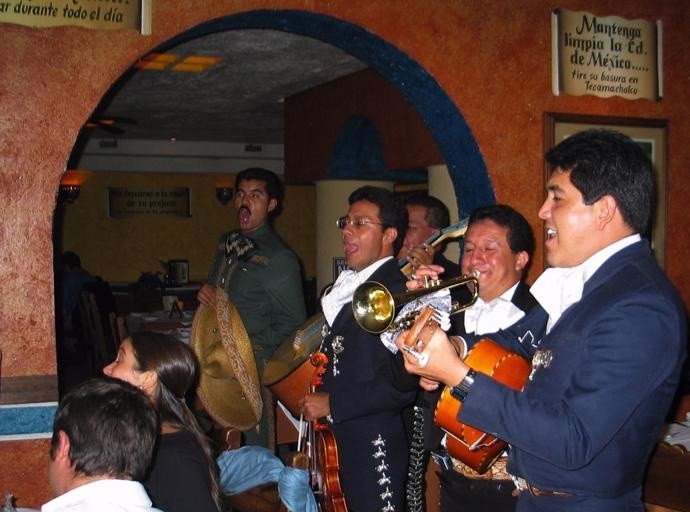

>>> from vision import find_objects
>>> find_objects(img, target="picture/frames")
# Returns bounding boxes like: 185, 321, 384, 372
544, 111, 669, 272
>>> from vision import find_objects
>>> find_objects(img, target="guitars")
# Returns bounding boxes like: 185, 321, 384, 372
401, 304, 532, 475
262, 216, 469, 421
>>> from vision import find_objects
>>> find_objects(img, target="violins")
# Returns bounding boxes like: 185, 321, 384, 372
307, 351, 347, 511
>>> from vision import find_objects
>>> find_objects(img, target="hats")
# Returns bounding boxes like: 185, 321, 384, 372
188, 286, 264, 430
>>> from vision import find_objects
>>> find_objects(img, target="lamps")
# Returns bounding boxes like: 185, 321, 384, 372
58, 170, 88, 205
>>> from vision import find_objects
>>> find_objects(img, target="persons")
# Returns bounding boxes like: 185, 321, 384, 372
298, 185, 427, 511
391, 127, 688, 511
197, 166, 306, 463
103, 327, 226, 512
398, 195, 461, 512
34, 374, 167, 512
409, 204, 548, 512
55, 250, 89, 314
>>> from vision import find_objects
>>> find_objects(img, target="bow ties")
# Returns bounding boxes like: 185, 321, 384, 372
225, 232, 257, 259
463, 297, 525, 335
321, 271, 359, 322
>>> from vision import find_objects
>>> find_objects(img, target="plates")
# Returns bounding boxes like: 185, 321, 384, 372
130, 312, 160, 321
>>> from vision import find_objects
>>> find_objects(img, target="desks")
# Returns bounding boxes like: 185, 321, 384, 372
140, 310, 194, 345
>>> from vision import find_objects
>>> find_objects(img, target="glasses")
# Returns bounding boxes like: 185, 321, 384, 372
336, 217, 394, 230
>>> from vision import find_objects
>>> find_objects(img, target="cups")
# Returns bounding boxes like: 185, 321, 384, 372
162, 296, 178, 314
182, 311, 193, 322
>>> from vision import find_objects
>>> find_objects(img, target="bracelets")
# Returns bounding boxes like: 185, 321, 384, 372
448, 368, 478, 403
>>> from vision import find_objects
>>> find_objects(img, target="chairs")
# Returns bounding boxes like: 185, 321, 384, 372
73, 287, 108, 375
220, 425, 308, 512
108, 311, 128, 347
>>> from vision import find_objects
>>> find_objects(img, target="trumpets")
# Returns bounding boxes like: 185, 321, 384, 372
352, 270, 479, 335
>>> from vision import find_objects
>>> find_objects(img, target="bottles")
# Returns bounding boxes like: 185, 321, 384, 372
158, 261, 175, 288
